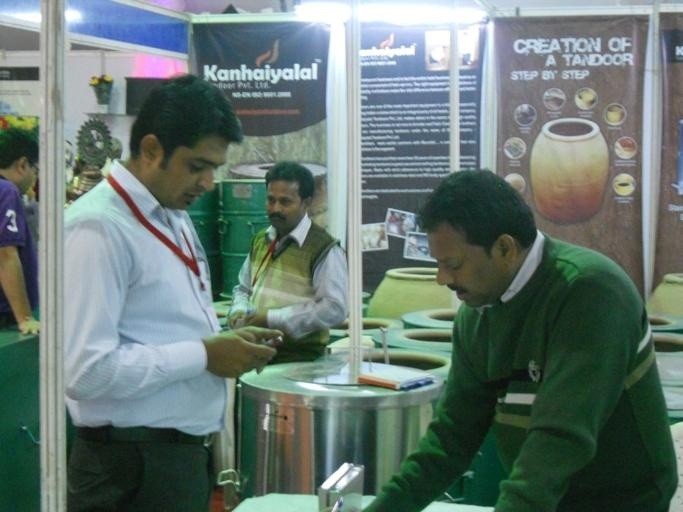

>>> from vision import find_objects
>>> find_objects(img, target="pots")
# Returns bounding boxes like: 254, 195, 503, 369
217, 362, 442, 499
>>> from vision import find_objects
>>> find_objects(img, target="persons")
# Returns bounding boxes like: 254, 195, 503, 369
229, 162, 350, 472
357, 169, 678, 512
0, 128, 41, 337
64, 74, 285, 512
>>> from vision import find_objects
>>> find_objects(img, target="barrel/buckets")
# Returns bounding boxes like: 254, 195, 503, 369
218, 178, 272, 297
186, 180, 218, 300
230, 159, 329, 233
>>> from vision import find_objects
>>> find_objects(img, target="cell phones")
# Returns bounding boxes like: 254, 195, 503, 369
257, 335, 284, 347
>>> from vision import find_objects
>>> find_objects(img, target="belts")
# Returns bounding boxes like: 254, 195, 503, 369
78, 427, 215, 447
